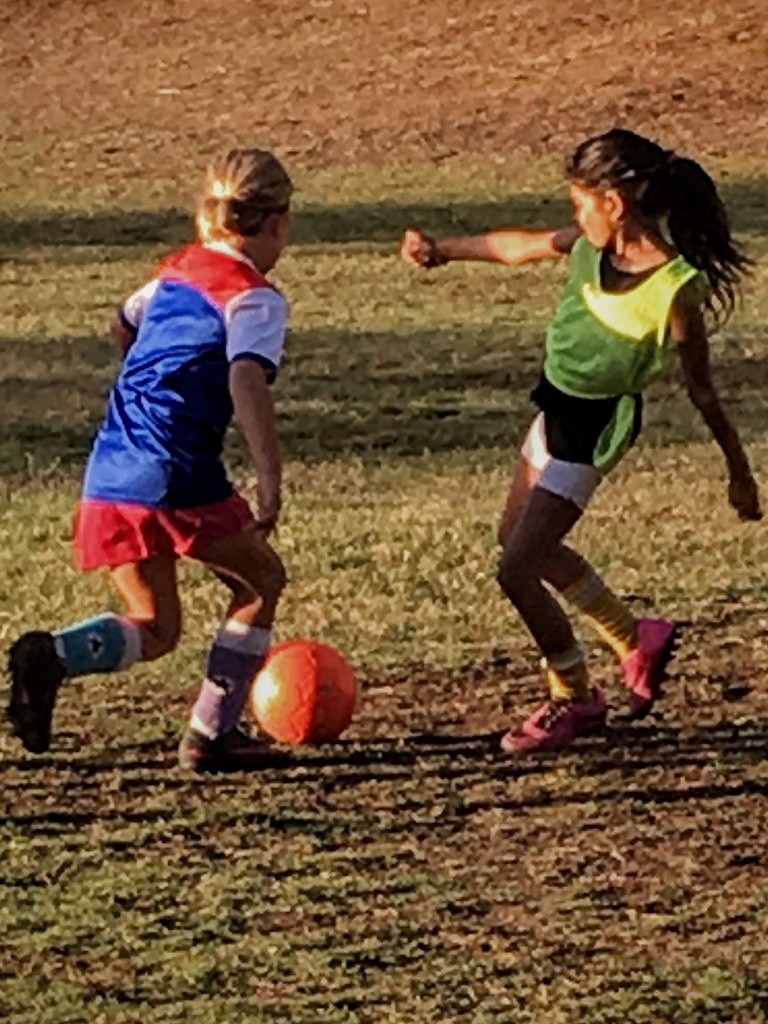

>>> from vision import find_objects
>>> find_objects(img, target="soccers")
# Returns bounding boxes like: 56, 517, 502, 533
246, 638, 357, 745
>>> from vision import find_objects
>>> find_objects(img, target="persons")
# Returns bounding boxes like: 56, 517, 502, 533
8, 146, 294, 771
398, 129, 762, 752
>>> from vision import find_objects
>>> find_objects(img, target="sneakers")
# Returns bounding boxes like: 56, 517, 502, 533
9, 631, 65, 755
180, 730, 294, 769
626, 620, 678, 722
503, 686, 607, 753
